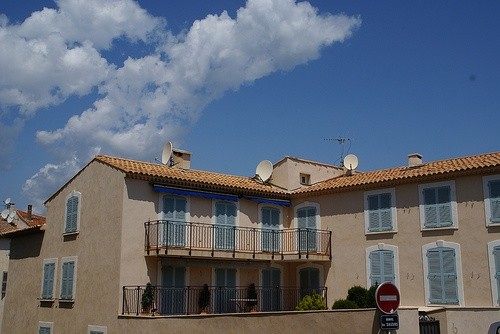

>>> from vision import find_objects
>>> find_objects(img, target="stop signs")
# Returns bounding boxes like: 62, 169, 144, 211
375, 281, 400, 314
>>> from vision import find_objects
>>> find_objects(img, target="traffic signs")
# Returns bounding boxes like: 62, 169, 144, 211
381, 314, 400, 330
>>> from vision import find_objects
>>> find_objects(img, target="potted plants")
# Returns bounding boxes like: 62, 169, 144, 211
247, 284, 259, 312
198, 284, 211, 315
141, 281, 154, 314
